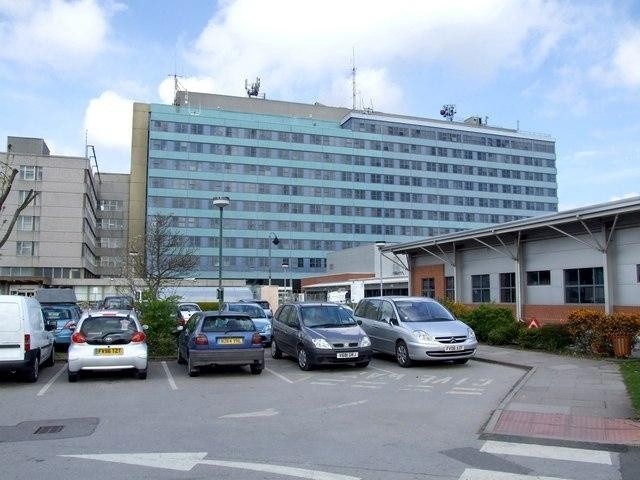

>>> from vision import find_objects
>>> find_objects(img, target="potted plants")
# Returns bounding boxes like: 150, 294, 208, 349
600, 309, 640, 357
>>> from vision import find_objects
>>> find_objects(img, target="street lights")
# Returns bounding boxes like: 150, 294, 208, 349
268, 231, 279, 286
212, 196, 231, 311
375, 240, 386, 296
282, 262, 288, 302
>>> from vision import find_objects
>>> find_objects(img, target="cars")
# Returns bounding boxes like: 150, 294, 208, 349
0, 296, 149, 383
176, 298, 273, 377
270, 301, 373, 372
351, 296, 479, 368
